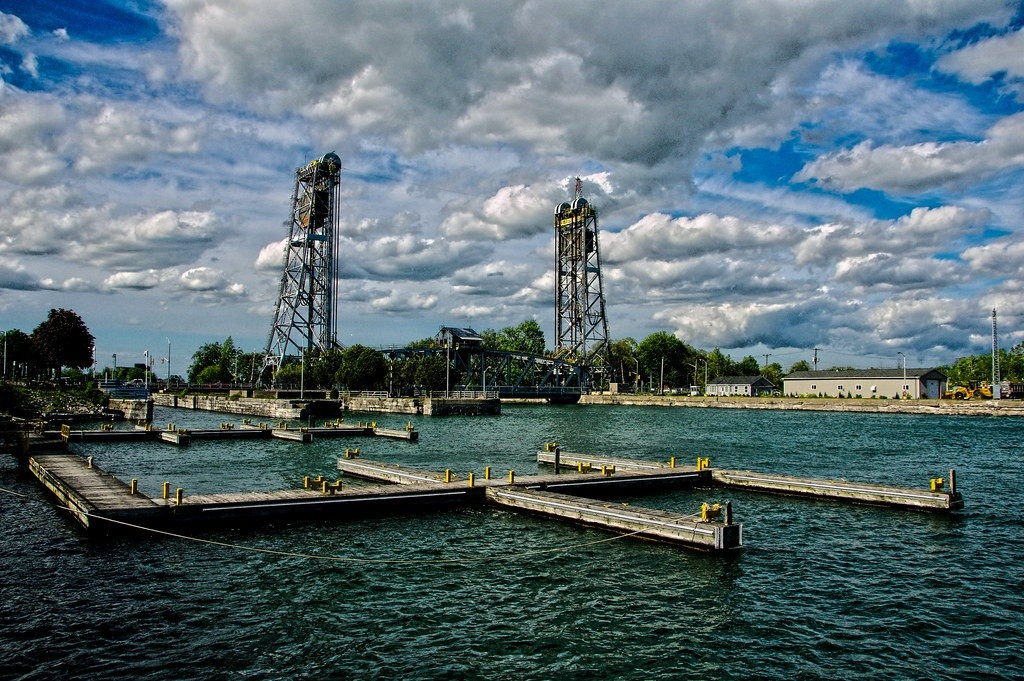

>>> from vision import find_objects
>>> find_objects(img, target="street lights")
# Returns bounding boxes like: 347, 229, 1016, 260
898, 351, 906, 392
144, 351, 148, 390
483, 365, 491, 399
699, 359, 707, 385
160, 358, 170, 389
112, 353, 117, 373
92, 345, 96, 379
0, 330, 6, 378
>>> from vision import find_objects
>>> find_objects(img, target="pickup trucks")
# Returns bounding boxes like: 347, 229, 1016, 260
124, 379, 151, 388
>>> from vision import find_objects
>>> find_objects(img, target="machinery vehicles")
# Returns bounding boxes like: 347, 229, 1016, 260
944, 380, 993, 400
